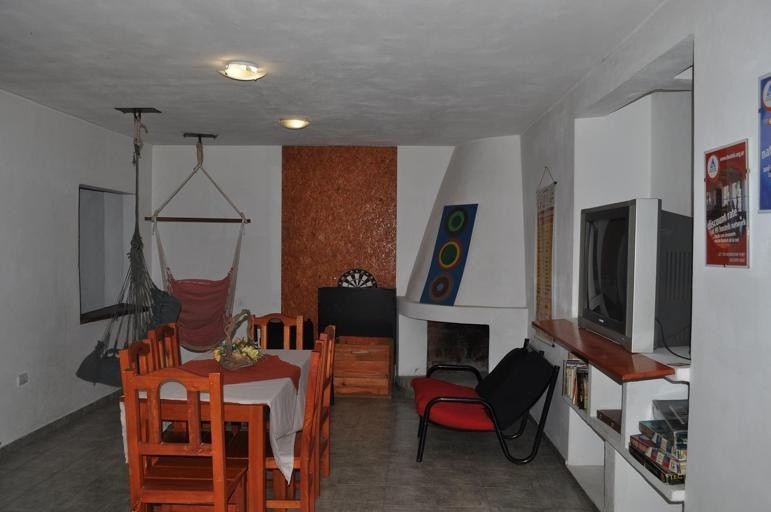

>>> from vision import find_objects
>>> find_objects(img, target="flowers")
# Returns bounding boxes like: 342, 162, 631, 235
212, 334, 265, 366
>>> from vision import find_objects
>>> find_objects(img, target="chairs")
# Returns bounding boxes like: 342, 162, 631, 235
227, 339, 327, 511
410, 340, 561, 466
246, 312, 303, 351
120, 369, 249, 511
149, 322, 181, 370
117, 331, 230, 491
315, 326, 336, 498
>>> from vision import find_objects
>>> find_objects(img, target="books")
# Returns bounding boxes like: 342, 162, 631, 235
562, 359, 587, 411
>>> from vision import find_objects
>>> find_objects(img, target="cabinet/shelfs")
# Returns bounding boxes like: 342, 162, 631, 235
527, 325, 690, 512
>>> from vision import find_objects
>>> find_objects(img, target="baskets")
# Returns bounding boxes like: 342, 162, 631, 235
213, 309, 266, 370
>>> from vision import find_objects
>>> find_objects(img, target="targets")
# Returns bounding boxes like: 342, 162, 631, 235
338, 269, 377, 287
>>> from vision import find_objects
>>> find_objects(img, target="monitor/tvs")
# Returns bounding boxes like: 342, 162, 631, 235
577, 198, 693, 353
